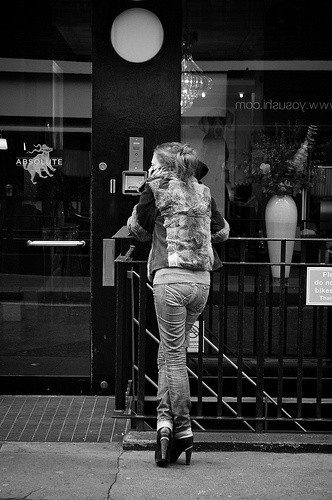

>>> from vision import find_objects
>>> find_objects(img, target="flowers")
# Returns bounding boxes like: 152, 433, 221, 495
240, 119, 324, 198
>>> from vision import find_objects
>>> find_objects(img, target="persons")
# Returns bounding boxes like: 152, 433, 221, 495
36, 149, 70, 259
128, 141, 230, 468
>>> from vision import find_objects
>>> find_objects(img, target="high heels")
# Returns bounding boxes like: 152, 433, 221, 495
169, 436, 194, 465
155, 427, 173, 467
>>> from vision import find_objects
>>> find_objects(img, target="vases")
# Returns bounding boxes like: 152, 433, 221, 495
265, 195, 296, 279
